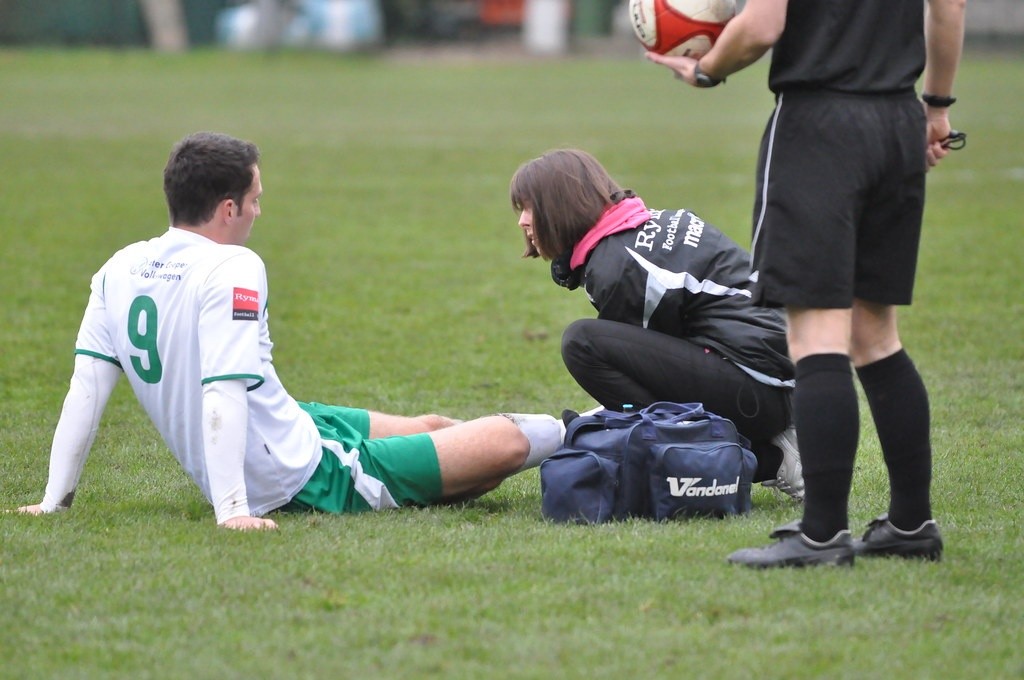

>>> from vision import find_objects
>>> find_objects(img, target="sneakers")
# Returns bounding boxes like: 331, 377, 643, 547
761, 425, 806, 510
851, 513, 945, 563
725, 518, 857, 571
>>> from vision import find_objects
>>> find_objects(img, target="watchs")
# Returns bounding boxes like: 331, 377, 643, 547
693, 61, 727, 89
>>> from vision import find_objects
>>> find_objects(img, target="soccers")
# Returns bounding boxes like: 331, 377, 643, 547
628, 0, 737, 68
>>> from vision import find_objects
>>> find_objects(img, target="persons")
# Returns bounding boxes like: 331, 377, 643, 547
1, 130, 605, 532
627, 0, 971, 573
509, 149, 804, 505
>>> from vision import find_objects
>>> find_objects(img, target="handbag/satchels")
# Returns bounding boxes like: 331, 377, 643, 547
539, 401, 758, 527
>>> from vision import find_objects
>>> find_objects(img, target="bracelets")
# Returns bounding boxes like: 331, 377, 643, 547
921, 94, 956, 108
